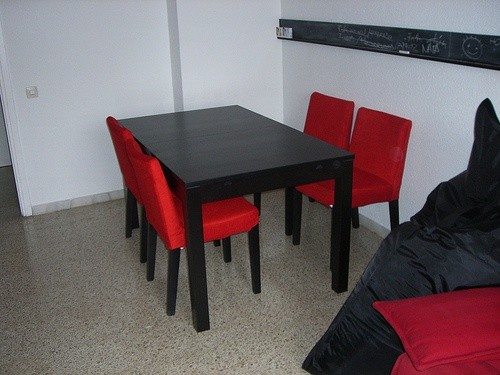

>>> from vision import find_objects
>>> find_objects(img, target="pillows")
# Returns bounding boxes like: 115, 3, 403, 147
372, 288, 500, 371
411, 97, 500, 234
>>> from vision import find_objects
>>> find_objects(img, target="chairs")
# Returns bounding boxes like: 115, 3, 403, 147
108, 92, 412, 334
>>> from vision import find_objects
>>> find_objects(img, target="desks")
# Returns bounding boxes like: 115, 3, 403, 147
118, 106, 356, 332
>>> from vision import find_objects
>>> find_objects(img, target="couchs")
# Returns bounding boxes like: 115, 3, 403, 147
301, 98, 500, 375
